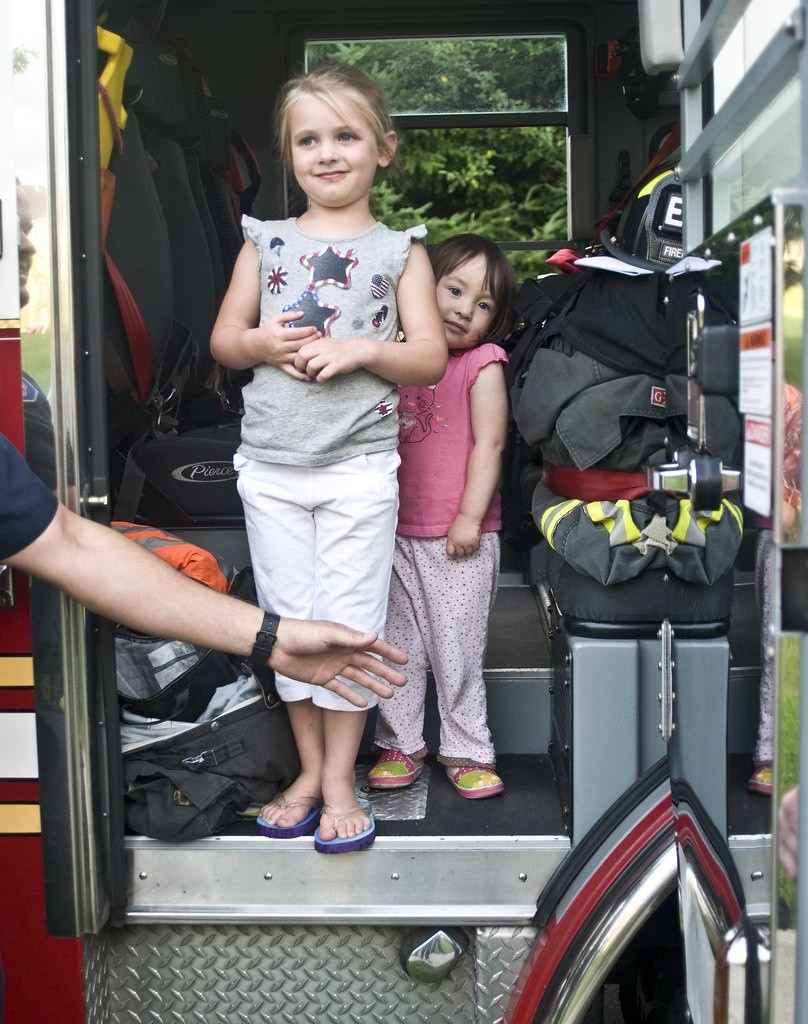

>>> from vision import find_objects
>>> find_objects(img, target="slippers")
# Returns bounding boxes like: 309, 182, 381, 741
313, 797, 377, 853
369, 750, 425, 788
255, 787, 324, 838
446, 767, 504, 799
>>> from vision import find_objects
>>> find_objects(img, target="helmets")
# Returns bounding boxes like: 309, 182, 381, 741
600, 162, 684, 272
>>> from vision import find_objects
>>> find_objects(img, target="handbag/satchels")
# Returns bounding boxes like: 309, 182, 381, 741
108, 520, 302, 843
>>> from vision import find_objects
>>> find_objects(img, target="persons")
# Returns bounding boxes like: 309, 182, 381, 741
778, 380, 802, 887
210, 65, 449, 855
752, 515, 782, 792
15, 178, 87, 936
0, 436, 410, 707
364, 233, 512, 798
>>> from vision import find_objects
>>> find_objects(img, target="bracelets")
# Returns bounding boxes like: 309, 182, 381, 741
248, 610, 282, 664
